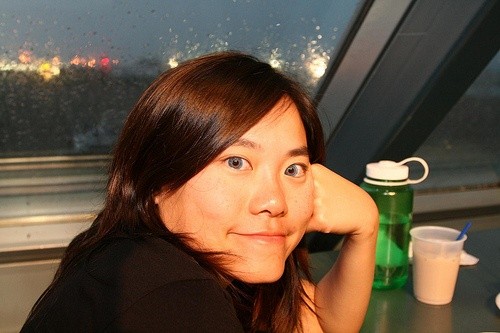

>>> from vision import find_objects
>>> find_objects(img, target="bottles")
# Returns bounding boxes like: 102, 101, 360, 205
360, 157, 429, 290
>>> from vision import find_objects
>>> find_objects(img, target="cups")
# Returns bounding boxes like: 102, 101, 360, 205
409, 226, 467, 305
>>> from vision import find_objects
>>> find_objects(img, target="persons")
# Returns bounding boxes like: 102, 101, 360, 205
21, 49, 380, 332
